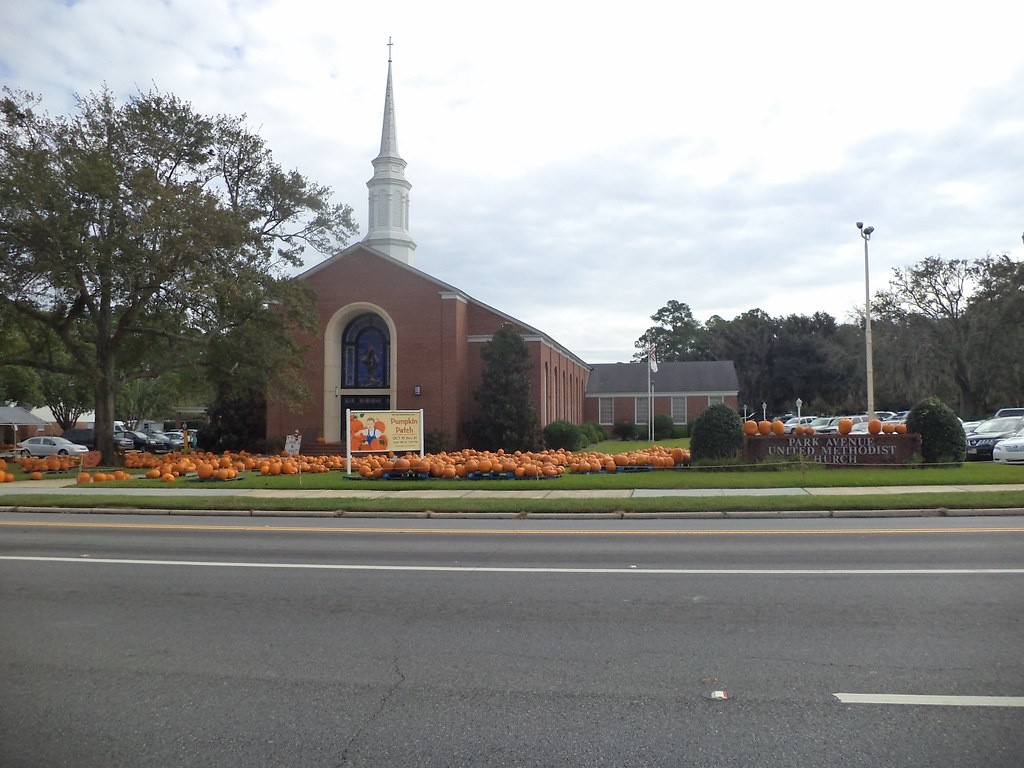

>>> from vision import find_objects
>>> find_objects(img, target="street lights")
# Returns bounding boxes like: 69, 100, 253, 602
857, 221, 876, 421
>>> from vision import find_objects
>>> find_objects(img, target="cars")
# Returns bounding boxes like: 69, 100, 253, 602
780, 406, 1024, 465
15, 435, 89, 459
61, 420, 198, 454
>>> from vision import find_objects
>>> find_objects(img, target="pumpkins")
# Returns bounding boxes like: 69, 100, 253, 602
0, 438, 690, 482
744, 419, 907, 435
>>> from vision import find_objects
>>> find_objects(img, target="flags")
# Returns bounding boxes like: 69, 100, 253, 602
651, 342, 658, 374
